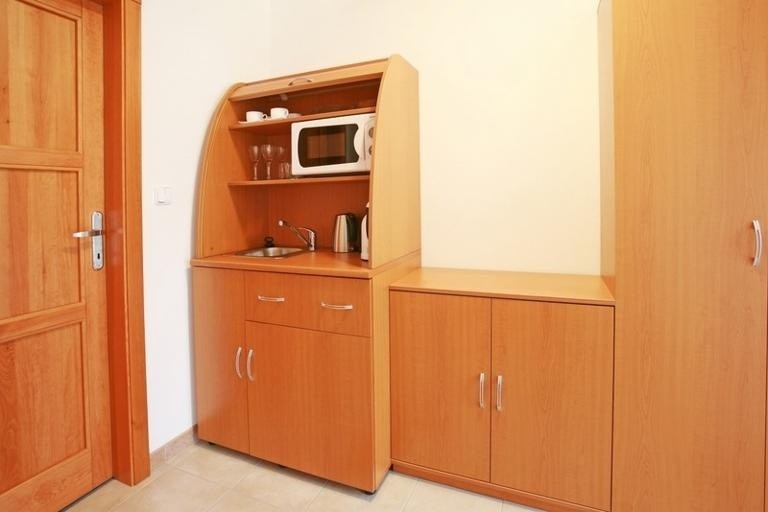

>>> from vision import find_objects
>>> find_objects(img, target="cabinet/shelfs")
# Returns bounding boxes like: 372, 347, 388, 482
390, 264, 615, 510
187, 51, 422, 497
595, 0, 768, 511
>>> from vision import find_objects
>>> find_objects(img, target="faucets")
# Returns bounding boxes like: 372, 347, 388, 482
279, 219, 316, 251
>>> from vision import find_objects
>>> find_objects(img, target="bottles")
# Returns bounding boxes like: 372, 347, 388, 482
333, 213, 356, 253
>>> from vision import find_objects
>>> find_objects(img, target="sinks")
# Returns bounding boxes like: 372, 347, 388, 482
236, 246, 309, 260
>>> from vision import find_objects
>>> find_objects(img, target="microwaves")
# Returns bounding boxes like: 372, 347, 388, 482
291, 113, 376, 177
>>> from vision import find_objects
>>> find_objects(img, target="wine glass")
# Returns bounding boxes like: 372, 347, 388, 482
247, 144, 289, 181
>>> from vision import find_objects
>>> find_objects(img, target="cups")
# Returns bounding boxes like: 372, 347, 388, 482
270, 107, 289, 119
246, 110, 268, 122
